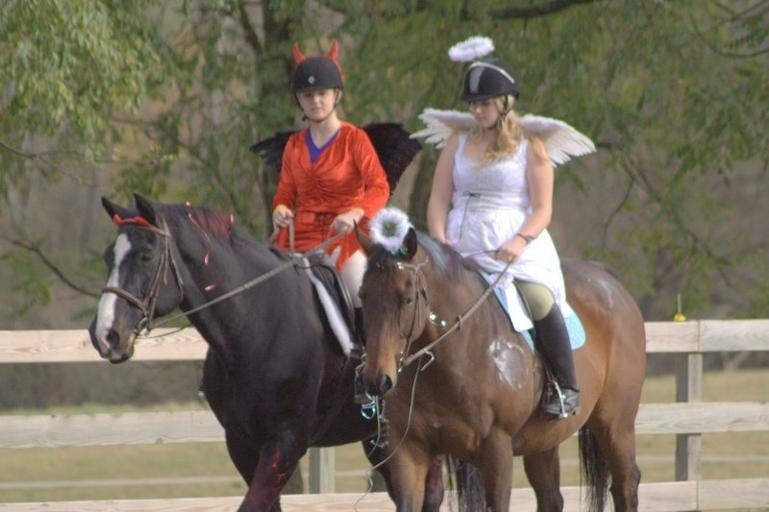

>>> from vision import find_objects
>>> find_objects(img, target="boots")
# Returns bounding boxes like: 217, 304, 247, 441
533, 303, 580, 415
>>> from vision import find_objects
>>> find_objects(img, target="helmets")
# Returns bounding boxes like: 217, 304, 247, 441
460, 57, 520, 102
290, 56, 345, 92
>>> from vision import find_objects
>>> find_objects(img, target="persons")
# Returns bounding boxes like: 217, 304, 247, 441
266, 40, 392, 351
424, 57, 582, 420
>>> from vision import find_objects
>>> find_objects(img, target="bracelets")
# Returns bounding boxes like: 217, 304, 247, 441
515, 231, 535, 243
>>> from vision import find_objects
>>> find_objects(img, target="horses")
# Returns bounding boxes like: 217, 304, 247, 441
352, 218, 647, 512
87, 191, 565, 512
